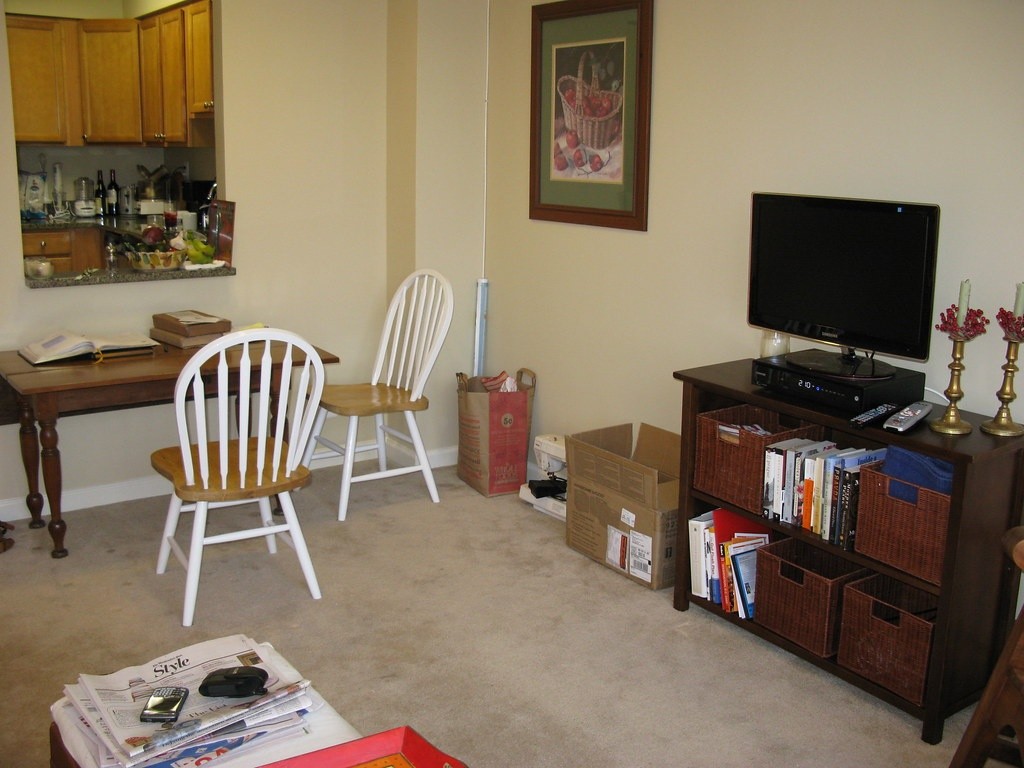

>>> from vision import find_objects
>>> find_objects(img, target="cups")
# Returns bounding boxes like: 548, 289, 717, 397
182, 213, 197, 232
164, 201, 176, 233
761, 330, 790, 358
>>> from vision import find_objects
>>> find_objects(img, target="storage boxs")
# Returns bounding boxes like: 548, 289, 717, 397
563, 420, 681, 592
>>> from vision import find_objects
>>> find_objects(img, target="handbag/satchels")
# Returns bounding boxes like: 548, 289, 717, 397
454, 368, 536, 499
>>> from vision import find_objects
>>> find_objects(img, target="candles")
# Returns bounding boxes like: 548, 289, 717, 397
1012, 282, 1024, 319
957, 279, 971, 328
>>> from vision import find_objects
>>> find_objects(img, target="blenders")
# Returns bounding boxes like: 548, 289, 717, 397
66, 177, 96, 217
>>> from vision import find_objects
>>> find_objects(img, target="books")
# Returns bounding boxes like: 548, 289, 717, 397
689, 507, 774, 620
18, 330, 162, 366
762, 438, 888, 550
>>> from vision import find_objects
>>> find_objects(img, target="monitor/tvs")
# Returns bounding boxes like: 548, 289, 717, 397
745, 191, 941, 380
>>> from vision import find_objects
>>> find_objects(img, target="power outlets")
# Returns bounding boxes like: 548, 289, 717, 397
182, 160, 189, 176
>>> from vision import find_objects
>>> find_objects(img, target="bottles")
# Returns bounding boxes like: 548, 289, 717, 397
106, 170, 120, 217
95, 171, 106, 218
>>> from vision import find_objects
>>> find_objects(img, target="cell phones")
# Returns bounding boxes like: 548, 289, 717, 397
141, 686, 189, 723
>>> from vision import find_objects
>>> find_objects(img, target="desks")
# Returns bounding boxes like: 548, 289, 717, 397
0, 340, 340, 560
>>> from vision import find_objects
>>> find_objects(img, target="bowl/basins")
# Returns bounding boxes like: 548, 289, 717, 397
125, 251, 188, 272
26, 262, 54, 280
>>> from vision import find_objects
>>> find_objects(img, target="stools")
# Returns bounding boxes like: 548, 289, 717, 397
947, 523, 1024, 768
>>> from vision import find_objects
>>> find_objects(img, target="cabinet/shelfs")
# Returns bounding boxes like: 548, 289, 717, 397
138, 6, 215, 148
182, 0, 215, 120
23, 226, 104, 274
6, 13, 84, 149
76, 17, 144, 148
672, 357, 1024, 747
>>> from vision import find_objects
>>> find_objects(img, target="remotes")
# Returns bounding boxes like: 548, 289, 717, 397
883, 401, 932, 433
847, 403, 901, 431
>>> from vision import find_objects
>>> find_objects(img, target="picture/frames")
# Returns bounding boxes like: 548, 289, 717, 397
529, 0, 655, 232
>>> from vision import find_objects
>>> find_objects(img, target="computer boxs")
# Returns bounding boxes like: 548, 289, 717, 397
752, 348, 926, 414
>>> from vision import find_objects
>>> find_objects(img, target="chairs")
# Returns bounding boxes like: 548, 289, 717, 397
151, 326, 323, 628
291, 267, 455, 523
